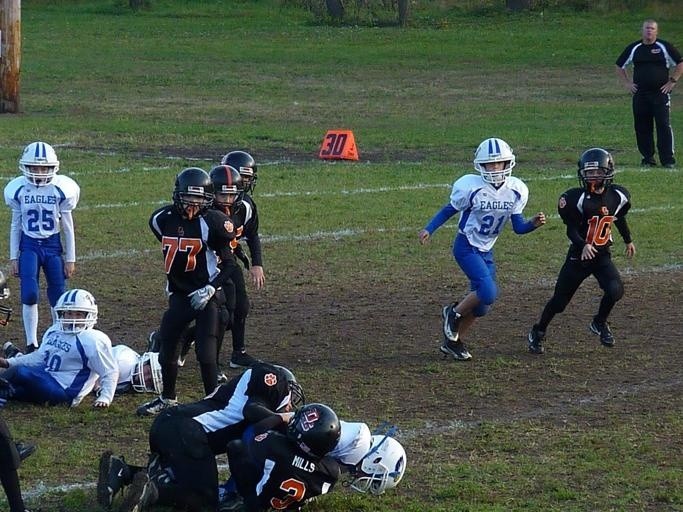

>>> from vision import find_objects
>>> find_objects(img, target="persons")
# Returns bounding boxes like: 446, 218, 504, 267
94, 361, 405, 512
147, 164, 246, 384
1, 270, 31, 512
1, 289, 120, 407
614, 18, 680, 169
135, 167, 233, 420
174, 148, 264, 370
3, 140, 80, 350
113, 343, 173, 393
528, 147, 635, 353
419, 136, 547, 361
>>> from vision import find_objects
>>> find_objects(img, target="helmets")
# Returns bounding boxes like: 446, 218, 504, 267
0, 272, 12, 325
130, 352, 163, 394
473, 138, 515, 184
577, 148, 614, 197
171, 151, 256, 222
54, 289, 98, 334
19, 142, 59, 186
271, 364, 406, 495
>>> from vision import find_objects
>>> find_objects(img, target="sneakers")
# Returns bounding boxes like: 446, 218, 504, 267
15, 443, 35, 460
98, 453, 130, 509
25, 344, 39, 355
137, 395, 178, 417
217, 368, 227, 383
527, 324, 544, 354
149, 331, 161, 352
440, 340, 472, 361
177, 341, 195, 367
441, 301, 463, 342
230, 351, 262, 369
116, 473, 158, 511
589, 318, 615, 346
3, 341, 23, 358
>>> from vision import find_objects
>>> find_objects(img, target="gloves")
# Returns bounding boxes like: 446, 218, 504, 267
187, 284, 215, 310
